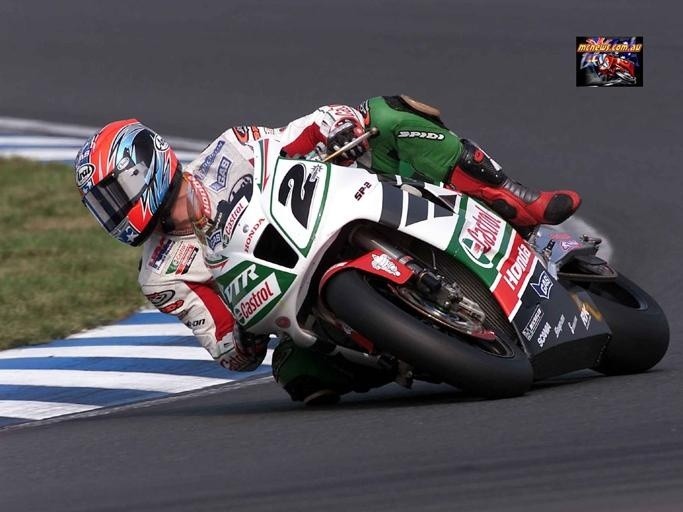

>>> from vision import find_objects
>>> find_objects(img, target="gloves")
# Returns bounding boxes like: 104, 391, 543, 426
324, 118, 371, 163
231, 321, 269, 364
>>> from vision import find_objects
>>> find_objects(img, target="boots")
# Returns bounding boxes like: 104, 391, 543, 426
443, 136, 584, 236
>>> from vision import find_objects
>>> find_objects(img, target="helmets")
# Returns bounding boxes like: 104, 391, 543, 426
68, 117, 183, 249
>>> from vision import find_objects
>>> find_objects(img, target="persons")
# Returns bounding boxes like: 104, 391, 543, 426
71, 93, 582, 409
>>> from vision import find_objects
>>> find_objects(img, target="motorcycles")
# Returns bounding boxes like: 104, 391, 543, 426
215, 128, 669, 398
